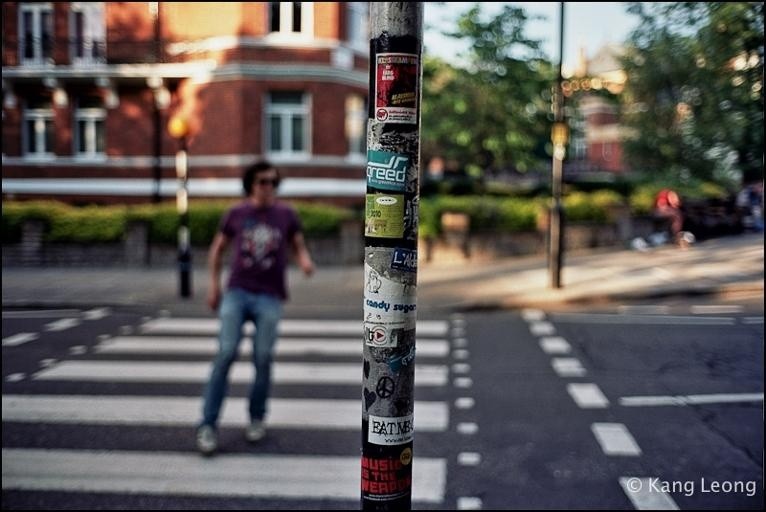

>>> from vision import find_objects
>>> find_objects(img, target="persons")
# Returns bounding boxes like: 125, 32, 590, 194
193, 160, 314, 456
735, 181, 765, 230
654, 187, 685, 241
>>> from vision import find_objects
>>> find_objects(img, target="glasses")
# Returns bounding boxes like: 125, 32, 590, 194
259, 177, 278, 187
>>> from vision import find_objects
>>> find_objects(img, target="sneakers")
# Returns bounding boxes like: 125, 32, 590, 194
197, 425, 218, 454
248, 421, 265, 441
